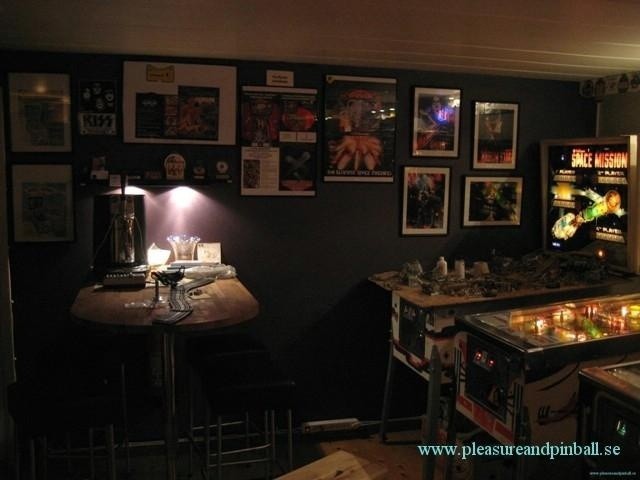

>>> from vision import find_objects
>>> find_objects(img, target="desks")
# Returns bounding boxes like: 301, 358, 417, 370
72, 260, 257, 480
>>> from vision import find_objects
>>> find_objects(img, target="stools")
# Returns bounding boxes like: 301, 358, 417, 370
187, 333, 299, 480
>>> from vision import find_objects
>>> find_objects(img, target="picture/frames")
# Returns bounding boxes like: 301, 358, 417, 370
121, 60, 399, 196
8, 69, 79, 249
400, 86, 523, 236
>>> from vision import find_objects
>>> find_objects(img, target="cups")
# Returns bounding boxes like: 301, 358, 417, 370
455, 259, 465, 280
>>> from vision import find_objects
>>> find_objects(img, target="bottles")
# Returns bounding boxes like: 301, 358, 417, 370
437, 256, 448, 277
